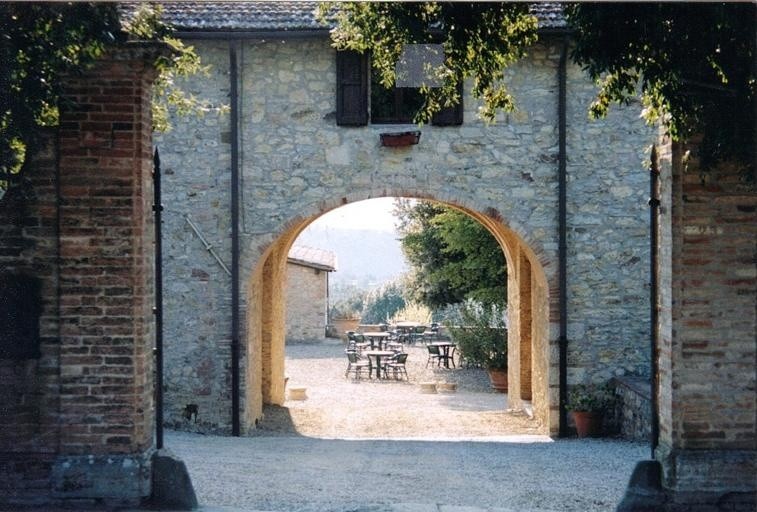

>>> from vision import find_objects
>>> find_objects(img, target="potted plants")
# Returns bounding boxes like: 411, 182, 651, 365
563, 382, 617, 437
443, 300, 507, 390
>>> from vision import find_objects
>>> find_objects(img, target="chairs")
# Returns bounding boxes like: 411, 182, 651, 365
344, 323, 457, 380
